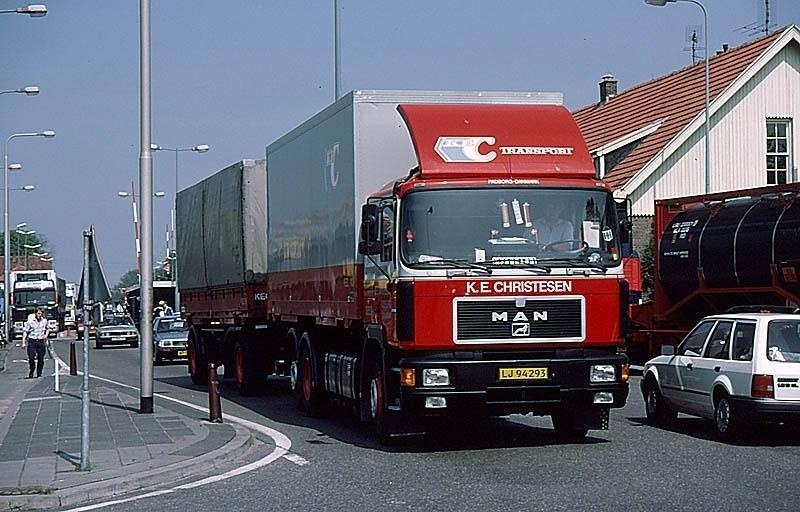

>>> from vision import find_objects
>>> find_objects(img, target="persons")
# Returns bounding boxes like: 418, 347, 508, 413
533, 200, 573, 253
163, 302, 173, 315
153, 301, 164, 317
21, 307, 50, 378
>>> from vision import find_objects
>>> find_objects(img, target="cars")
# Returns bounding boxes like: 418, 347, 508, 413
96, 314, 140, 348
638, 307, 799, 439
151, 314, 188, 365
78, 314, 97, 339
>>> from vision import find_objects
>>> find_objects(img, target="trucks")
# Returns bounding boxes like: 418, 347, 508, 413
64, 284, 80, 327
620, 183, 800, 361
12, 271, 65, 339
170, 88, 633, 452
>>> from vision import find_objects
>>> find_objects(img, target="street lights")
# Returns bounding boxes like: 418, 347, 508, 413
1, 86, 41, 97
119, 191, 166, 200
152, 142, 211, 312
1, 4, 50, 18
3, 125, 55, 339
644, 0, 712, 194
